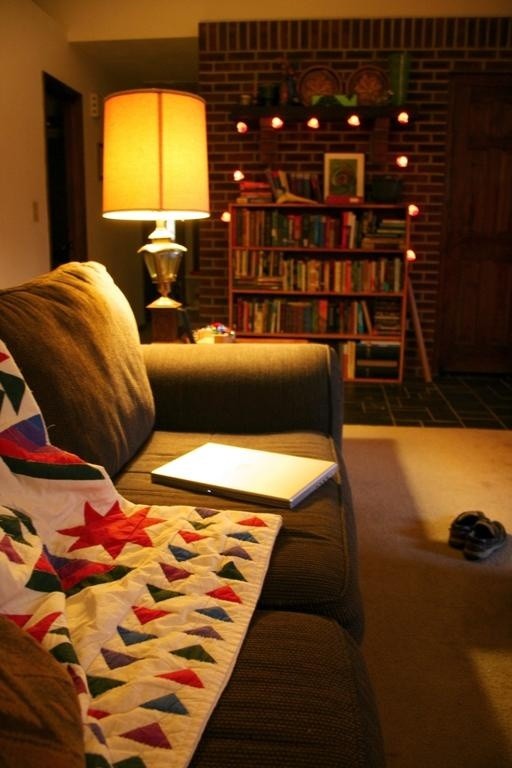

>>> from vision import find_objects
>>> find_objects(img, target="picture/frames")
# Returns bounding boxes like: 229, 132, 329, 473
324, 152, 365, 203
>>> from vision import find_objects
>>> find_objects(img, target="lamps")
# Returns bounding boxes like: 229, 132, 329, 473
102, 89, 211, 339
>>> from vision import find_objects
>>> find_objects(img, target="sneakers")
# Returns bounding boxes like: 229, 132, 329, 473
449, 509, 484, 550
464, 519, 507, 561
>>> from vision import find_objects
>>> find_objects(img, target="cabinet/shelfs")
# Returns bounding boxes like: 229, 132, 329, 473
228, 203, 410, 384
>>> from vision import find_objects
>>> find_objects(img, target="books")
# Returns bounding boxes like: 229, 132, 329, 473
195, 320, 236, 344
228, 164, 408, 381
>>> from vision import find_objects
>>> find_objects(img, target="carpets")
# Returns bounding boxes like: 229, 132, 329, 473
341, 424, 512, 768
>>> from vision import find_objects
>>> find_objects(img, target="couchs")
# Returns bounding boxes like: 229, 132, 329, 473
0, 261, 386, 768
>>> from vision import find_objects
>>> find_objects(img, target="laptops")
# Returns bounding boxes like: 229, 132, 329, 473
151, 441, 342, 509
